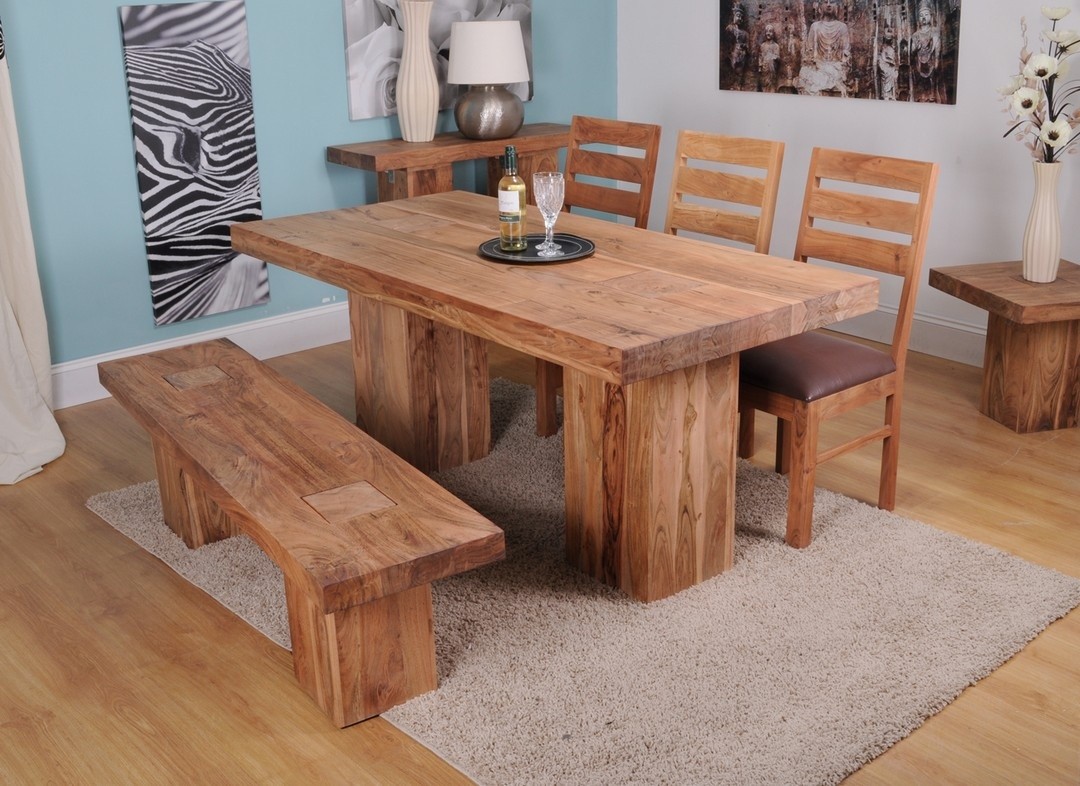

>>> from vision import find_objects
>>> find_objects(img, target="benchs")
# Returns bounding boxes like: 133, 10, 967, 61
98, 337, 505, 731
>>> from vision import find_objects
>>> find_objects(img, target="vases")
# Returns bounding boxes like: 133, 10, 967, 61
1024, 160, 1061, 283
396, 0, 439, 142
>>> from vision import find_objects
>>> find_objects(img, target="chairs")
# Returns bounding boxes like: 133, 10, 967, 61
538, 115, 940, 551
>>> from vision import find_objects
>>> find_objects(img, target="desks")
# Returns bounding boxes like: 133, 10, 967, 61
230, 190, 878, 601
928, 260, 1080, 433
326, 123, 578, 206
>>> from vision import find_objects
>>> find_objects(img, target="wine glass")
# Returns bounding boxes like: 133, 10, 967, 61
534, 176, 565, 257
531, 171, 563, 250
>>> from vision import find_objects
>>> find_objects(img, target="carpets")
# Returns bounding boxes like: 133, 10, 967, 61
85, 378, 1080, 786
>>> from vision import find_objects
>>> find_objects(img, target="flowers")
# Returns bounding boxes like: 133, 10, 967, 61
999, 6, 1080, 161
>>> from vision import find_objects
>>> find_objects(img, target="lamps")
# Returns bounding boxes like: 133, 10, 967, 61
446, 19, 531, 137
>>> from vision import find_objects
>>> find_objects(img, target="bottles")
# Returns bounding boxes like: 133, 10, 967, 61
497, 144, 528, 252
